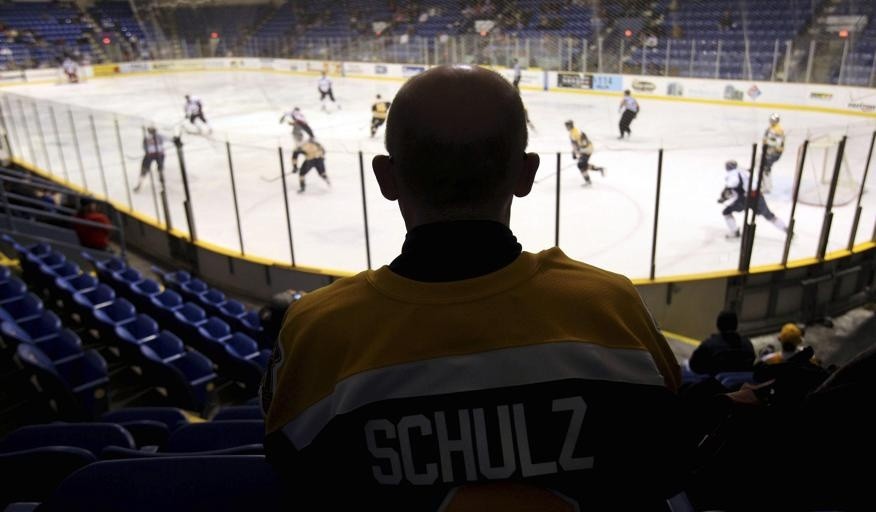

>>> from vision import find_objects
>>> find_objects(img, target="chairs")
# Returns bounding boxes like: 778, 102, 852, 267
2, 2, 872, 88
0, 155, 876, 511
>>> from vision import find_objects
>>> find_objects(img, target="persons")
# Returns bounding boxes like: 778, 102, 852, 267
183, 93, 209, 131
290, 133, 333, 195
513, 56, 521, 86
0, 1, 137, 83
759, 317, 807, 366
12, 168, 58, 221
564, 117, 606, 186
716, 9, 736, 32
280, 106, 305, 132
564, 1, 681, 76
316, 70, 338, 109
524, 107, 535, 133
616, 90, 640, 141
445, 0, 569, 64
133, 125, 178, 194
756, 115, 786, 183
70, 196, 111, 248
137, 1, 445, 57
715, 160, 793, 243
364, 93, 390, 137
687, 309, 755, 372
256, 62, 679, 512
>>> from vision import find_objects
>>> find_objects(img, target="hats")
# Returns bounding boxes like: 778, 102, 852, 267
781, 323, 800, 345
